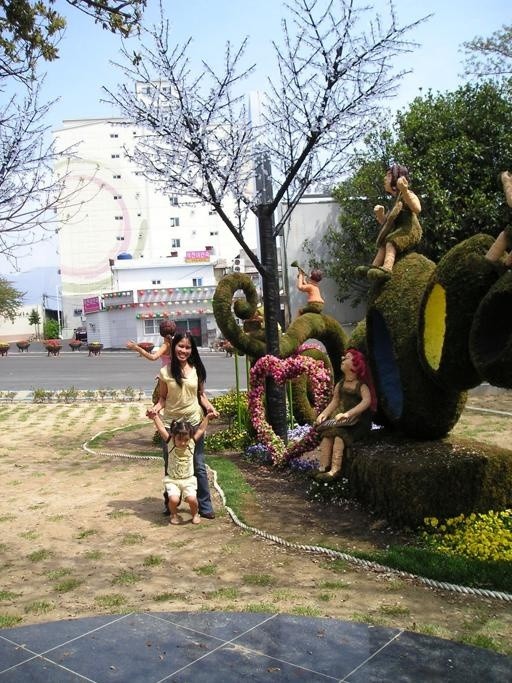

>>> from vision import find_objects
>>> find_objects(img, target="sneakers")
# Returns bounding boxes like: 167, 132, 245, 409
169, 515, 181, 524
191, 515, 201, 524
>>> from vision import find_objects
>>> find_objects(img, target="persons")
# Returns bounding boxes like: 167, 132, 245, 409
297, 270, 325, 315
466, 166, 512, 274
353, 164, 424, 282
146, 407, 220, 525
309, 347, 379, 482
126, 320, 177, 445
148, 331, 217, 519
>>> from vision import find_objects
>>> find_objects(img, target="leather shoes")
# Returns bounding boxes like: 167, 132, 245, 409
202, 512, 215, 518
162, 508, 169, 515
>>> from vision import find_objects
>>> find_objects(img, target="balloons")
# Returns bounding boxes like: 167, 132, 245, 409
101, 287, 215, 319
